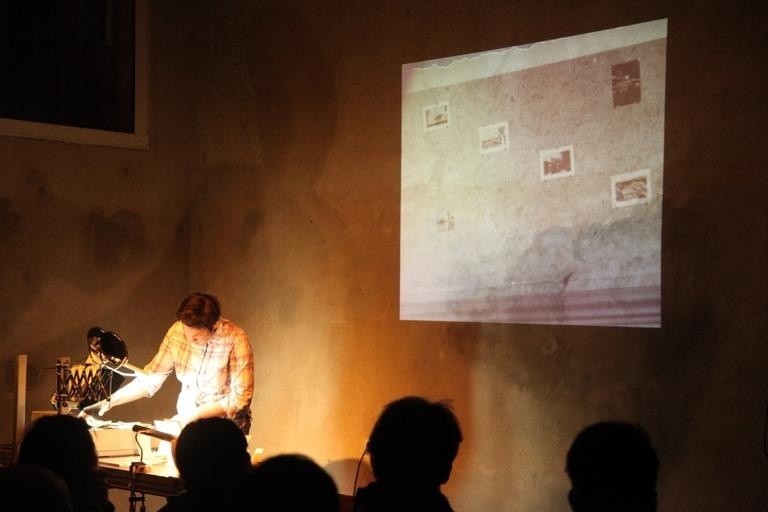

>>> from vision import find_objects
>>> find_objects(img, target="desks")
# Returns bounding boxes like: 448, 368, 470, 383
100, 450, 187, 497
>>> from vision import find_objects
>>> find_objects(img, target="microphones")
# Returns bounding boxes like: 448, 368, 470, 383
129, 428, 156, 473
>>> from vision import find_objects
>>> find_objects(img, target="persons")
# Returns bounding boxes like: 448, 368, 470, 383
78, 291, 254, 436
12, 412, 116, 511
155, 416, 255, 511
253, 452, 341, 512
563, 420, 660, 511
353, 394, 463, 512
